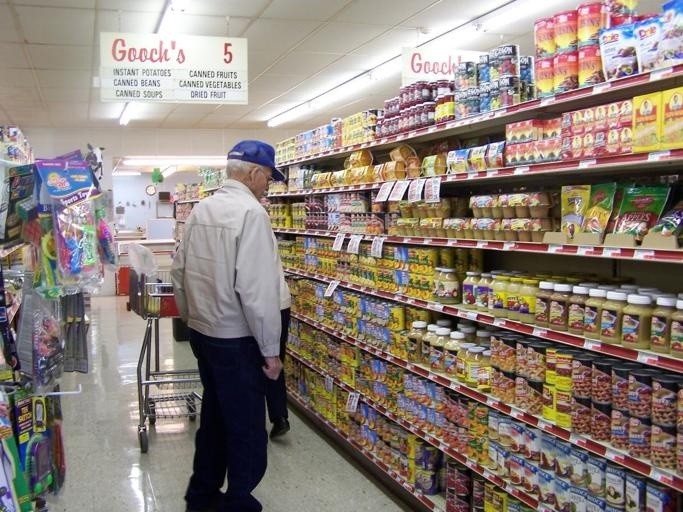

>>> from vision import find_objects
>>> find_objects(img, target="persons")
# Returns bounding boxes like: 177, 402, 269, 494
170, 139, 287, 512
249, 230, 290, 436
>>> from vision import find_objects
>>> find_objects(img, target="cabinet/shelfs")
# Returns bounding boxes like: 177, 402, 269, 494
0, 157, 38, 512
174, 1, 682, 512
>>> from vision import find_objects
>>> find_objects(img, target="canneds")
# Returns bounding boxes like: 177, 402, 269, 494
269, 0, 683, 512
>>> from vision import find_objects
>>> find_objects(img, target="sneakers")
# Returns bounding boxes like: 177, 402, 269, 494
269, 417, 290, 438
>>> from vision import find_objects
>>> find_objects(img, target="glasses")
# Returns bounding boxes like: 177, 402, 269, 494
260, 168, 276, 186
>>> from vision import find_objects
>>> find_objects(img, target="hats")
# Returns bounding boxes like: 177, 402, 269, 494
227, 140, 287, 181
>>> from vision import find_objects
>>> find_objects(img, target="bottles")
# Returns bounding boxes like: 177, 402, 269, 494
431, 327, 490, 393
409, 266, 683, 361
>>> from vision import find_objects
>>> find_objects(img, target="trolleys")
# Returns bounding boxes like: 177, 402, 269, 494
127, 269, 203, 452
